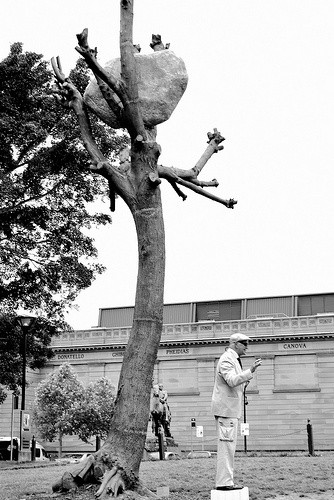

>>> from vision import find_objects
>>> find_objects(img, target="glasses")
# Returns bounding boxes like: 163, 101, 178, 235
238, 341, 247, 346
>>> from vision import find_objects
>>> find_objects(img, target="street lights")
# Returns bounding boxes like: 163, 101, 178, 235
10, 393, 19, 461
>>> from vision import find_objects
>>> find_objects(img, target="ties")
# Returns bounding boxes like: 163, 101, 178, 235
238, 358, 242, 369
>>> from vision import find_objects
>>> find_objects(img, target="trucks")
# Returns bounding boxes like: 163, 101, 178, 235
1, 436, 50, 461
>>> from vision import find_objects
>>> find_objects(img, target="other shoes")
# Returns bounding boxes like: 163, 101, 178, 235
216, 485, 244, 490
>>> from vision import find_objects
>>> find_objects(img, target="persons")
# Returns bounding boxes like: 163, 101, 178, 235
158, 383, 172, 423
207, 333, 263, 491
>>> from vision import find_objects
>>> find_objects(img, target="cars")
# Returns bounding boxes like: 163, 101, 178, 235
187, 451, 216, 459
55, 453, 93, 463
151, 452, 180, 460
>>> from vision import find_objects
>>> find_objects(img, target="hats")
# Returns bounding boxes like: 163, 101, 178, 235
230, 333, 253, 343
